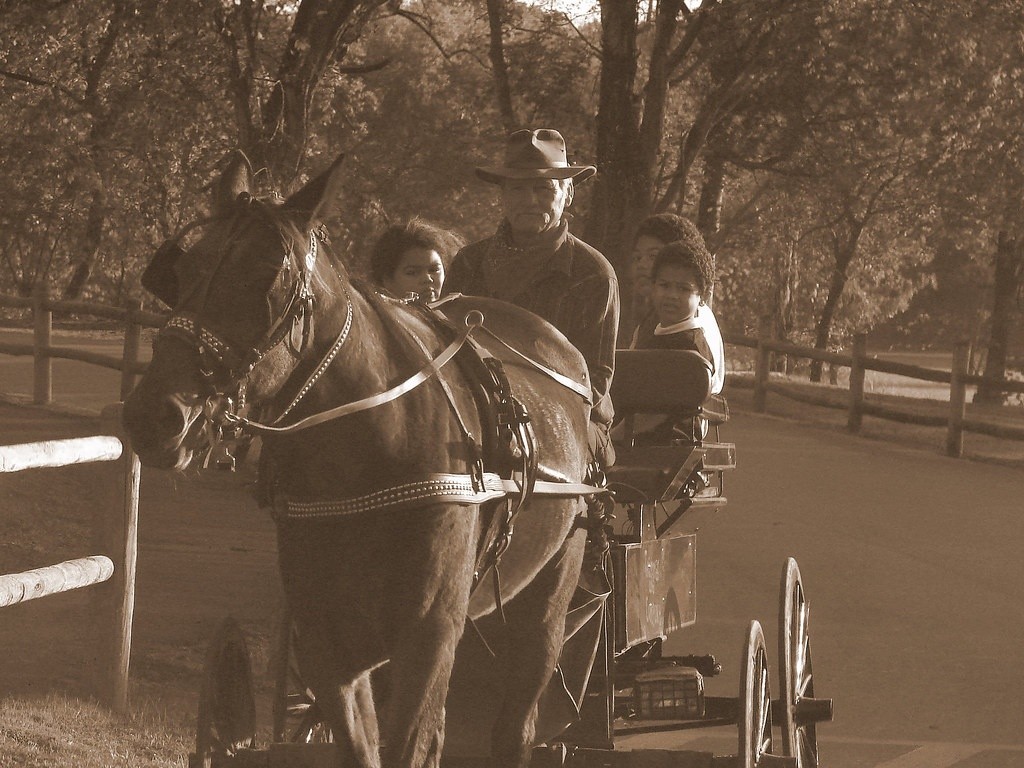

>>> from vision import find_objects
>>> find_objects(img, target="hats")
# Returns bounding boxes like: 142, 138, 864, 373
474, 127, 597, 188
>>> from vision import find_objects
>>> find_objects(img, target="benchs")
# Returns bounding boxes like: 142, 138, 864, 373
610, 349, 739, 508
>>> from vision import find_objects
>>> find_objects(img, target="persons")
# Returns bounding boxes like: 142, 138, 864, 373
438, 129, 616, 468
606, 213, 725, 486
371, 221, 465, 304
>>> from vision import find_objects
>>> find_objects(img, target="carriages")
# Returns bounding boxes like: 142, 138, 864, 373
119, 146, 836, 768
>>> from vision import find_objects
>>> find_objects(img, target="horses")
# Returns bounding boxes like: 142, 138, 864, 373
120, 147, 590, 768
